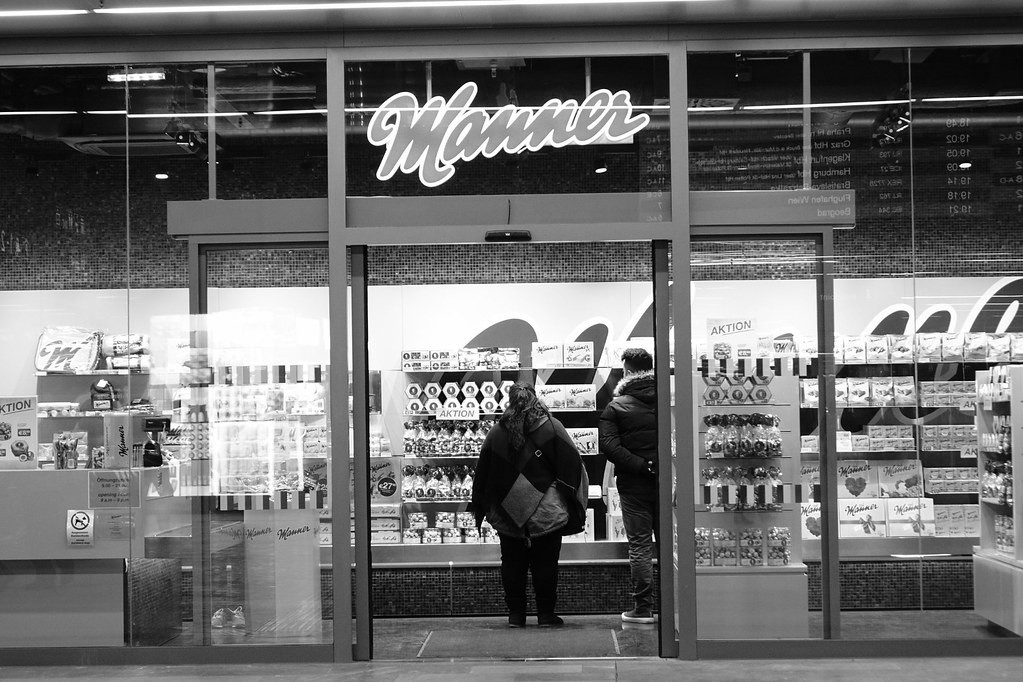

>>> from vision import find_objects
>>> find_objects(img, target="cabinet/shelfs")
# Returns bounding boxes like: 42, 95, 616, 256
175, 361, 1023, 640
35, 370, 149, 466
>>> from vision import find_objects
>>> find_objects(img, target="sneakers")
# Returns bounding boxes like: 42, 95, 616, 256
211, 607, 227, 628
227, 606, 245, 628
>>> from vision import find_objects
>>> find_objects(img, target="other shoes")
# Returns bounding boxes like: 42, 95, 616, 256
538, 614, 565, 629
621, 609, 660, 624
508, 616, 527, 629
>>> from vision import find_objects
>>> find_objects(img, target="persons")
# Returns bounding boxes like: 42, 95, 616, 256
600, 348, 659, 623
473, 381, 581, 629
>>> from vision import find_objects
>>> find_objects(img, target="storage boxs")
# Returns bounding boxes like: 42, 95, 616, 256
303, 332, 979, 546
